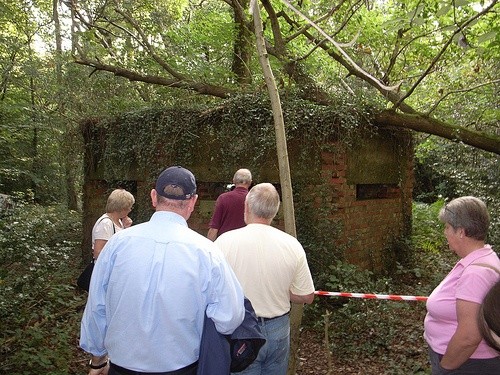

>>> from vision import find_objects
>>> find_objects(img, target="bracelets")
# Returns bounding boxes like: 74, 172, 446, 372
88, 358, 109, 369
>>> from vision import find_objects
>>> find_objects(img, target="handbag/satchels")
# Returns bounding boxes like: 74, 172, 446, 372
76, 262, 95, 292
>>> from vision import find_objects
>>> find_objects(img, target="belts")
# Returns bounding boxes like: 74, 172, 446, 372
256, 311, 290, 323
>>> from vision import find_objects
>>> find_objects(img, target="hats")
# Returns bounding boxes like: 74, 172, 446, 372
156, 166, 196, 200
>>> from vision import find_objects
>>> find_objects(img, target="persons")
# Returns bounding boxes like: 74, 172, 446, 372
81, 166, 245, 375
91, 188, 135, 262
207, 168, 253, 241
214, 182, 315, 375
423, 196, 500, 375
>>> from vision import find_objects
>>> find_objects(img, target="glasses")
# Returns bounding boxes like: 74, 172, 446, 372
128, 206, 134, 211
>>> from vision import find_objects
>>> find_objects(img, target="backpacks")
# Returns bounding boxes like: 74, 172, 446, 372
479, 277, 500, 354
